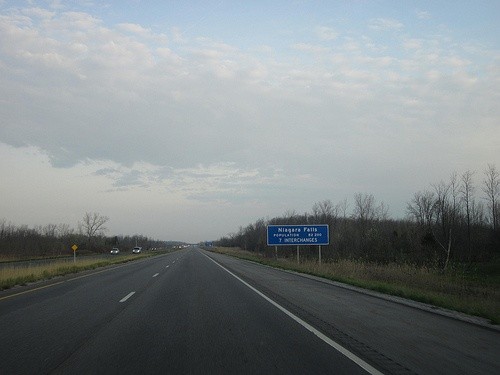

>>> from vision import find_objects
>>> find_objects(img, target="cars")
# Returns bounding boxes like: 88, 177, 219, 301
133, 247, 141, 254
110, 247, 119, 255
